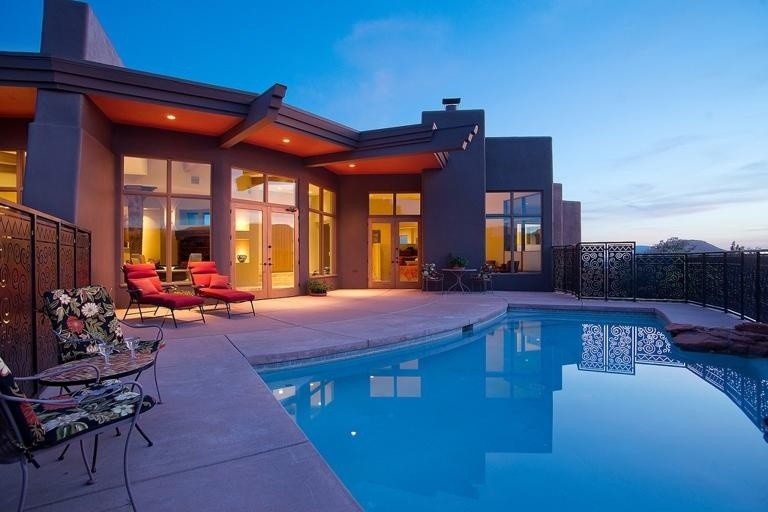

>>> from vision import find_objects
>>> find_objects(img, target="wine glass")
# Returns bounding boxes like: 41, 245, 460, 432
123, 336, 140, 359
99, 343, 115, 368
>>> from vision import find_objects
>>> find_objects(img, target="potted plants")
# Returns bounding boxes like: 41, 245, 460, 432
306, 280, 330, 296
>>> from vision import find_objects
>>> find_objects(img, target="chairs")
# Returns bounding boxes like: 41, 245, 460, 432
421, 255, 496, 297
123, 261, 256, 329
0, 284, 165, 511
